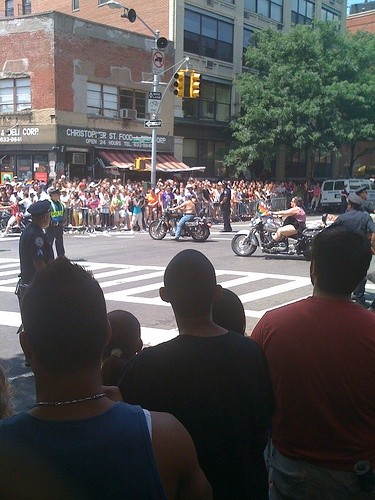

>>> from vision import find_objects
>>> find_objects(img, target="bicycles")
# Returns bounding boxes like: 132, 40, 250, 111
0, 212, 32, 233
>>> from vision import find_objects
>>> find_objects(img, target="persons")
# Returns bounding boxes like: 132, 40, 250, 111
15, 199, 56, 367
211, 284, 248, 338
98, 309, 142, 393
44, 188, 67, 259
0, 366, 16, 417
0, 256, 216, 500
326, 185, 375, 308
265, 197, 307, 250
168, 194, 196, 241
0, 176, 151, 236
119, 249, 271, 500
218, 180, 233, 232
251, 224, 375, 500
146, 176, 323, 228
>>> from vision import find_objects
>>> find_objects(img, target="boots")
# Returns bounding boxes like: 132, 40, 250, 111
261, 239, 278, 249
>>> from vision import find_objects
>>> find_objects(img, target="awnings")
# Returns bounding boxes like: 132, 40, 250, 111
97, 151, 205, 174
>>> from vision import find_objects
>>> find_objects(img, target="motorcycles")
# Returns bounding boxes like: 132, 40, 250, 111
148, 204, 212, 243
230, 209, 328, 262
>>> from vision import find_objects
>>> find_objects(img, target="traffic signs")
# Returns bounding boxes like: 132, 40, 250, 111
143, 119, 163, 128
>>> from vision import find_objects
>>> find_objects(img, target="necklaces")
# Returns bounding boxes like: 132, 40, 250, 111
34, 392, 109, 407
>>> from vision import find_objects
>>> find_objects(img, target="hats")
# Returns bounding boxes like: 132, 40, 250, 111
348, 193, 363, 206
27, 199, 51, 215
355, 185, 368, 195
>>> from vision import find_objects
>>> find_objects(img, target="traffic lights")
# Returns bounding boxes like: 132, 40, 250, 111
174, 72, 185, 99
190, 72, 202, 100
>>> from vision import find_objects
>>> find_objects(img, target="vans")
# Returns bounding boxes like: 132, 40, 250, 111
319, 178, 375, 207
